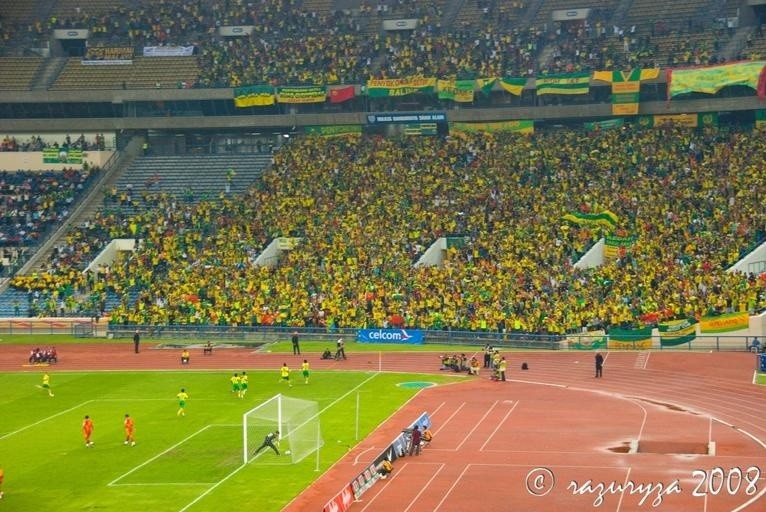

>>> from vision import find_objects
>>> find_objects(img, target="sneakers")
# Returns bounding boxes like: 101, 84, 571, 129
124, 440, 136, 447
85, 441, 95, 448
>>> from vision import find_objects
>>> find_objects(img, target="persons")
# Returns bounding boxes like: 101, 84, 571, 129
179, 350, 190, 364
320, 348, 333, 360
33, 370, 55, 398
80, 415, 95, 448
132, 328, 141, 354
439, 343, 508, 382
254, 431, 283, 457
123, 413, 136, 447
378, 457, 394, 480
292, 330, 301, 355
203, 340, 213, 355
0, 464, 4, 500
748, 336, 762, 354
278, 361, 293, 388
28, 346, 58, 365
407, 424, 423, 456
300, 359, 311, 384
334, 335, 347, 360
418, 426, 433, 446
238, 372, 248, 398
230, 373, 240, 393
176, 388, 188, 418
594, 351, 603, 378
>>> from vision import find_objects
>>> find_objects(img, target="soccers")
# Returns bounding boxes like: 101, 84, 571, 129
285, 451, 291, 455
